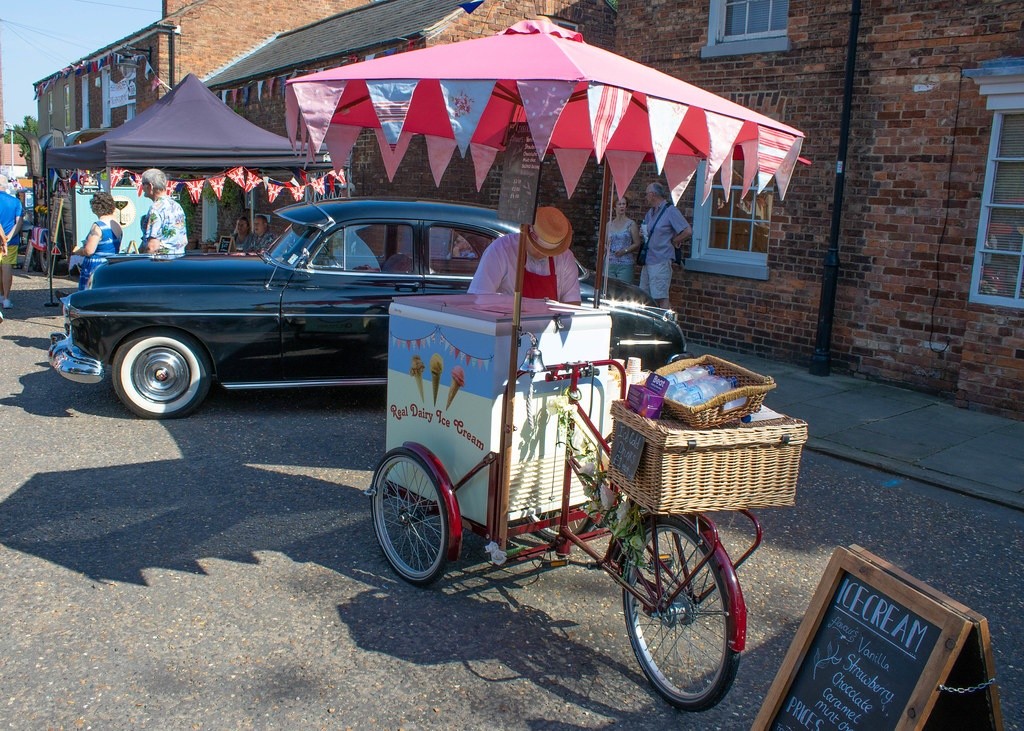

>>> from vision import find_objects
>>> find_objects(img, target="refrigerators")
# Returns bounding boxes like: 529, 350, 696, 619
385, 290, 612, 527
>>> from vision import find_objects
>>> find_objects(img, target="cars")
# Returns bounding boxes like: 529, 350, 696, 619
46, 200, 693, 422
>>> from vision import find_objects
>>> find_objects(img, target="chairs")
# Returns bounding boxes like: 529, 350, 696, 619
381, 253, 414, 273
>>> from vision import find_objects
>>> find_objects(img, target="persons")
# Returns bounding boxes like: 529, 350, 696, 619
74, 191, 123, 291
603, 196, 641, 285
142, 168, 188, 253
467, 206, 582, 305
246, 214, 278, 251
639, 182, 693, 308
0, 174, 23, 308
231, 217, 251, 247
0, 224, 7, 323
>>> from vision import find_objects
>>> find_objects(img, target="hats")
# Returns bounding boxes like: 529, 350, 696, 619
526, 206, 572, 257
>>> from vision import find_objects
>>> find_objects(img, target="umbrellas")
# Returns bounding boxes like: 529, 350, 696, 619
284, 17, 805, 310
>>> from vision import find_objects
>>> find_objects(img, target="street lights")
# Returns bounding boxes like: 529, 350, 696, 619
5, 123, 15, 180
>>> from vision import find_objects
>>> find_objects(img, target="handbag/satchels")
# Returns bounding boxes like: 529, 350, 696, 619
636, 241, 649, 266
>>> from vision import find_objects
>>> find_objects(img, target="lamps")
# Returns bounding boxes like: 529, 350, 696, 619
120, 47, 153, 80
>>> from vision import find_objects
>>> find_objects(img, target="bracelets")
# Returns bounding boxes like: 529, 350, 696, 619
625, 248, 628, 253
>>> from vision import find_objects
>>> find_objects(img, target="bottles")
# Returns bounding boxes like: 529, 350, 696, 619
661, 364, 752, 423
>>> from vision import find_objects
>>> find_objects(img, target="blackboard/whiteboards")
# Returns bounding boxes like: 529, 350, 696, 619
750, 544, 1002, 731
217, 235, 237, 254
50, 197, 65, 244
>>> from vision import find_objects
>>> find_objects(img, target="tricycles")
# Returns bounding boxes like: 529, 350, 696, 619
371, 290, 762, 713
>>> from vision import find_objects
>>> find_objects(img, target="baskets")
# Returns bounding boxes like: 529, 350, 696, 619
607, 398, 809, 514
642, 353, 777, 430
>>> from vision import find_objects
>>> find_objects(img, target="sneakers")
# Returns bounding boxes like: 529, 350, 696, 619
0, 295, 13, 308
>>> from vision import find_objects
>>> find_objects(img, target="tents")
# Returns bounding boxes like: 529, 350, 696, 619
45, 74, 352, 307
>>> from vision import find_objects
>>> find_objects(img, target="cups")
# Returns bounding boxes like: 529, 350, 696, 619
611, 356, 642, 384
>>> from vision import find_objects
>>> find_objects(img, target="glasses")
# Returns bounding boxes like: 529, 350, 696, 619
644, 192, 648, 196
90, 199, 94, 204
